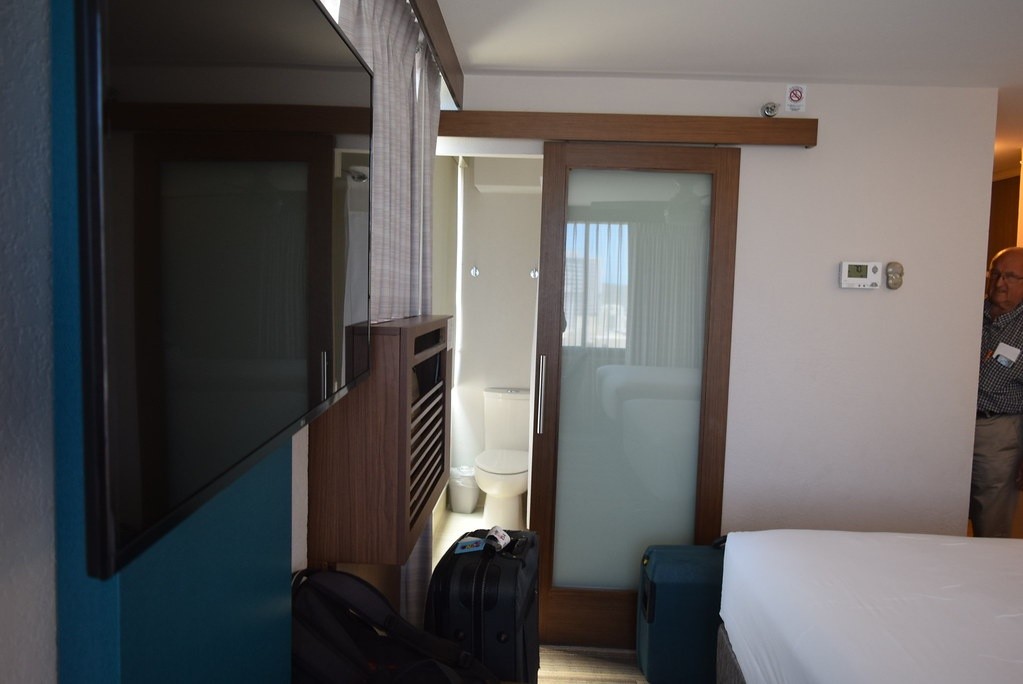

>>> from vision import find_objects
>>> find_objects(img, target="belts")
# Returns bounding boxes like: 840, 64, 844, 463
977, 410, 997, 419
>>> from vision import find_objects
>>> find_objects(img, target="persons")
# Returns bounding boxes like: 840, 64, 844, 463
969, 246, 1023, 538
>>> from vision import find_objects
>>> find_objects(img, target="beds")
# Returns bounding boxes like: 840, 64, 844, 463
621, 399, 695, 538
716, 528, 1023, 684
595, 365, 701, 438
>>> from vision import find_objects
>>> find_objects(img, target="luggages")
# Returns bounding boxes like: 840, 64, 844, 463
424, 531, 540, 684
636, 536, 724, 684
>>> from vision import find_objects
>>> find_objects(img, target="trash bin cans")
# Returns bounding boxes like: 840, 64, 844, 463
449, 466, 480, 514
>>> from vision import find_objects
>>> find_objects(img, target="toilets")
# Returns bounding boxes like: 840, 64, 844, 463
473, 388, 530, 530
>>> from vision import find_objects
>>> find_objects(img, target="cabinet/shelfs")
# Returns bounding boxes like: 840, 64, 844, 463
309, 317, 452, 566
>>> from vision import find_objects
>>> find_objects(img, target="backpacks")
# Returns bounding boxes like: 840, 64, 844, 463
290, 568, 499, 684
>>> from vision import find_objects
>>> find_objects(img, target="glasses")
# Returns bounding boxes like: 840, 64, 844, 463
988, 271, 1023, 280
